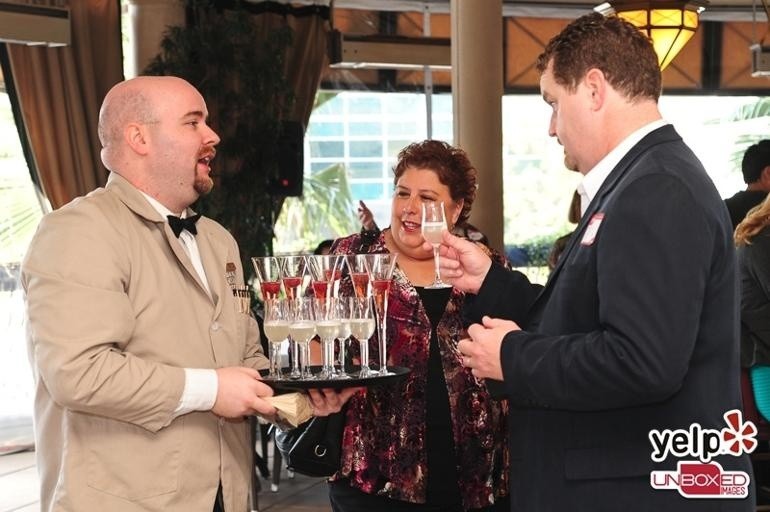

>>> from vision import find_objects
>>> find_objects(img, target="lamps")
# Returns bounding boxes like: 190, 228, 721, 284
592, 1, 708, 74
748, 1, 770, 79
325, 1, 453, 70
1, 1, 72, 52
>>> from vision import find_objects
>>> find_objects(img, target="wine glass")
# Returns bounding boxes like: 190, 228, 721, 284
250, 253, 398, 380
421, 200, 454, 290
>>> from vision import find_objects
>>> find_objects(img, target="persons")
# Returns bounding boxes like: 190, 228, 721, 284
724, 137, 770, 232
21, 76, 363, 512
327, 139, 511, 511
732, 192, 770, 427
546, 191, 581, 270
435, 11, 757, 512
314, 199, 378, 255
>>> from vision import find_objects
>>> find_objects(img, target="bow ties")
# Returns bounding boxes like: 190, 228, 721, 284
167, 213, 202, 238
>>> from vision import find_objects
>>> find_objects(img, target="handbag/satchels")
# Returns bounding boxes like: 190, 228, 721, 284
275, 404, 348, 477
